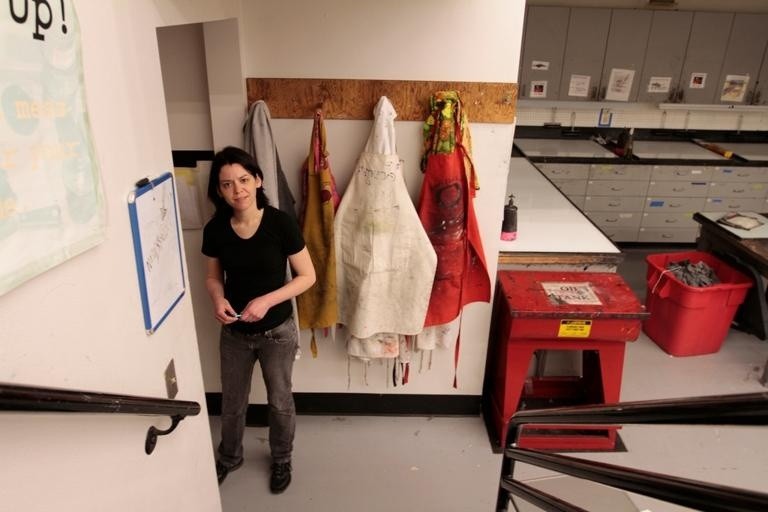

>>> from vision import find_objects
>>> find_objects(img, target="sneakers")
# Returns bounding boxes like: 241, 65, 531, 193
270, 463, 292, 494
216, 456, 244, 485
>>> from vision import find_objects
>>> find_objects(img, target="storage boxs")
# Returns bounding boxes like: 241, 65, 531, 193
643, 250, 753, 358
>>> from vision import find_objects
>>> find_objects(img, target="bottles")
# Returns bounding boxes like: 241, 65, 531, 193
615, 124, 635, 160
499, 193, 518, 241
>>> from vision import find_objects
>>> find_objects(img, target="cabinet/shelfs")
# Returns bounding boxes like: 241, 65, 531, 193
532, 161, 768, 250
518, 6, 768, 108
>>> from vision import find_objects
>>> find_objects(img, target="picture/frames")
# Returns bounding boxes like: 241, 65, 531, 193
0, 0, 109, 297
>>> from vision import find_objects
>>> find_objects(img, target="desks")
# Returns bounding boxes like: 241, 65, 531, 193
480, 267, 654, 452
484, 141, 626, 270
692, 209, 766, 344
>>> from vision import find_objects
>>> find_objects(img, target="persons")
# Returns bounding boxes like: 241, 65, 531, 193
198, 144, 319, 494
